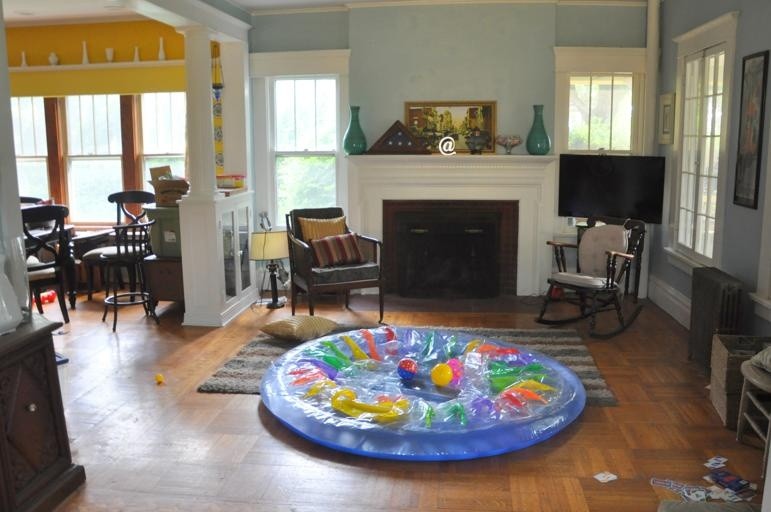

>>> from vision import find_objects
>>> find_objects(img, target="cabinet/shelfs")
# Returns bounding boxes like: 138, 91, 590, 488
0, 316, 86, 511
175, 187, 258, 327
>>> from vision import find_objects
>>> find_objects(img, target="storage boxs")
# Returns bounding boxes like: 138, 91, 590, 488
147, 207, 182, 257
148, 167, 188, 206
711, 335, 771, 428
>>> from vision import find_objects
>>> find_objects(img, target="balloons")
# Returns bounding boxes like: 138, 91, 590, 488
155, 373, 164, 383
397, 358, 418, 380
447, 358, 464, 382
430, 363, 453, 386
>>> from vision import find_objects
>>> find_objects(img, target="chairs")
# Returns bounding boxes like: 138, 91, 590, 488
534, 216, 645, 341
736, 343, 771, 479
19, 191, 155, 332
286, 208, 383, 323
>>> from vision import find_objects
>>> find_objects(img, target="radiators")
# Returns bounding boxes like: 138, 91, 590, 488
687, 266, 746, 363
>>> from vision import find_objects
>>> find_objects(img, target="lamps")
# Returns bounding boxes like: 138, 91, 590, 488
249, 231, 289, 308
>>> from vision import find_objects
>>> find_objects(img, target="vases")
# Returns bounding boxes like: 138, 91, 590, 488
104, 36, 166, 63
19, 40, 90, 66
343, 104, 366, 155
526, 104, 551, 155
0, 254, 23, 335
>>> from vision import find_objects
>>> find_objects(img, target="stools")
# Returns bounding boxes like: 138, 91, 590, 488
145, 255, 183, 316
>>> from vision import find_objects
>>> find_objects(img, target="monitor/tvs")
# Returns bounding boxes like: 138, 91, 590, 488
558, 154, 665, 225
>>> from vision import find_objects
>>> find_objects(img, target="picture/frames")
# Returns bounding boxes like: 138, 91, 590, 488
404, 101, 497, 153
657, 94, 674, 144
733, 50, 768, 210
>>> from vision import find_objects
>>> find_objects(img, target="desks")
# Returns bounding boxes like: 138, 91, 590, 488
575, 221, 647, 304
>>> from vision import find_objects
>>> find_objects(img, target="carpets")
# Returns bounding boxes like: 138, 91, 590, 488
196, 326, 617, 409
658, 499, 760, 512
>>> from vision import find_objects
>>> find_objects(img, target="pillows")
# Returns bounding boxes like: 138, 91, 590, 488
752, 344, 771, 374
260, 314, 339, 342
310, 232, 367, 269
297, 215, 347, 243
